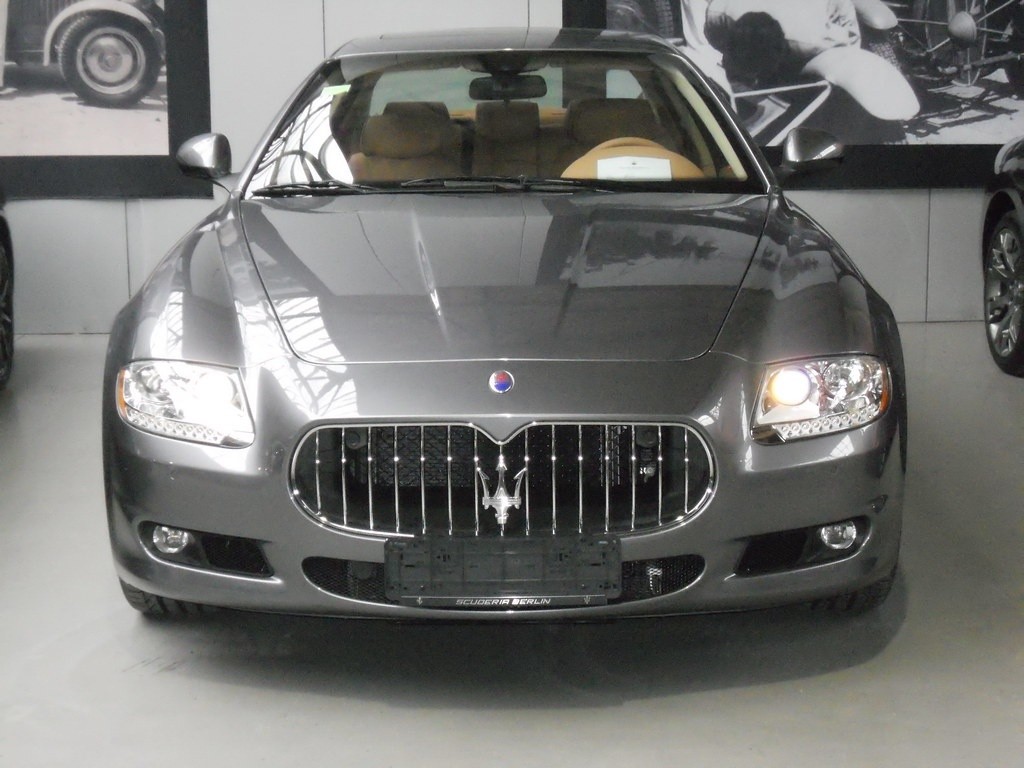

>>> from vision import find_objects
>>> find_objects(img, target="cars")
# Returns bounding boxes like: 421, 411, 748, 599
977, 137, 1024, 375
97, 21, 909, 623
0, 0, 166, 108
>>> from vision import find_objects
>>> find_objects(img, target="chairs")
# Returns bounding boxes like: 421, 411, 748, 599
342, 99, 676, 187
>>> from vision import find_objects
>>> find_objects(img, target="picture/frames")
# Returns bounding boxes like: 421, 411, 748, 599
561, 0, 1024, 190
0, 0, 216, 200
